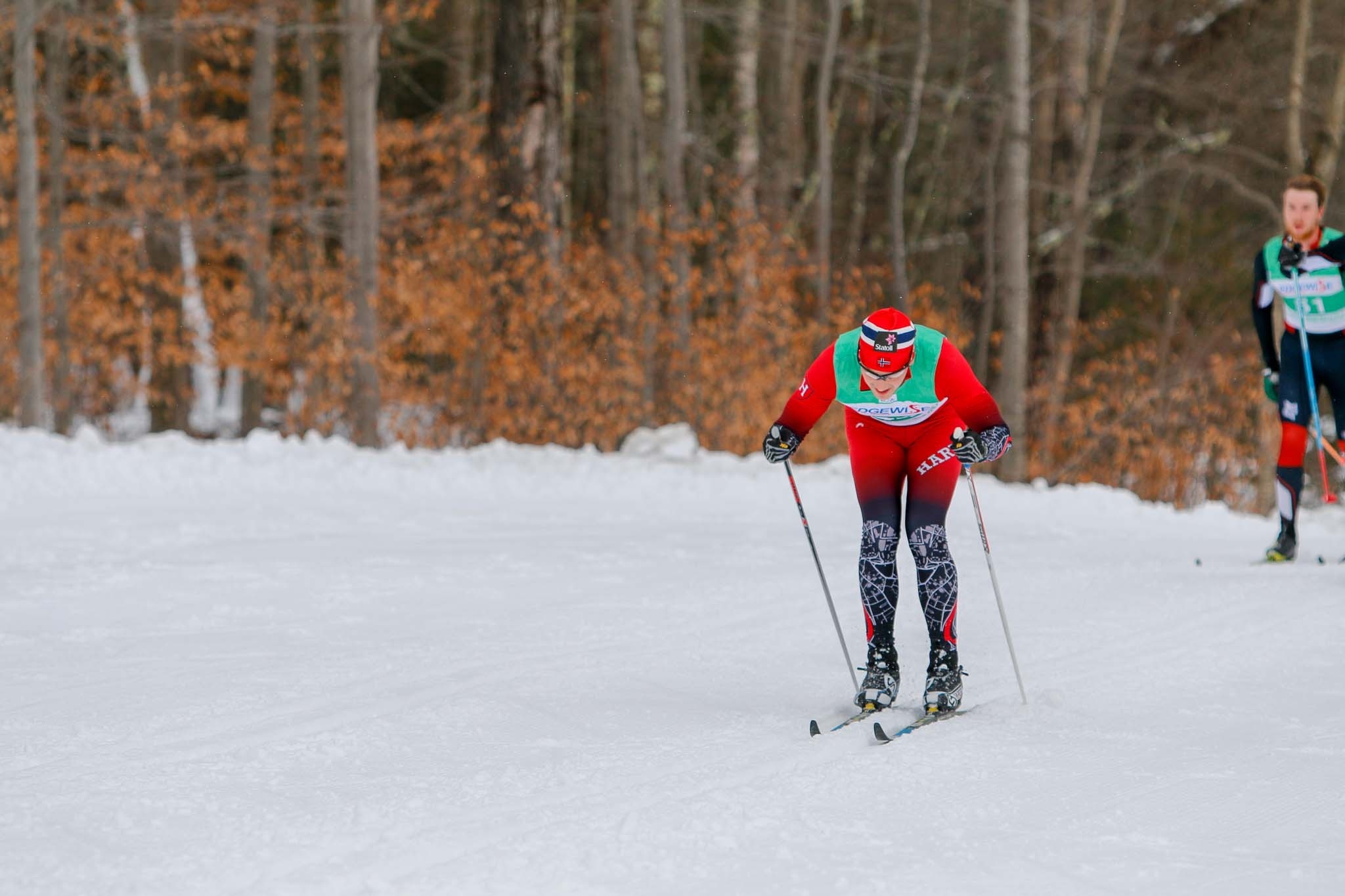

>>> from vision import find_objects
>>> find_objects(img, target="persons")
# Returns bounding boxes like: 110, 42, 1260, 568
1251, 173, 1345, 561
762, 307, 1012, 713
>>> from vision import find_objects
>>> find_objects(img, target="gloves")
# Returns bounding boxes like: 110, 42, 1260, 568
1262, 368, 1279, 403
1279, 243, 1305, 278
951, 431, 988, 465
763, 420, 802, 463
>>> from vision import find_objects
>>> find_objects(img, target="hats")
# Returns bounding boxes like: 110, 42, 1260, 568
858, 308, 916, 373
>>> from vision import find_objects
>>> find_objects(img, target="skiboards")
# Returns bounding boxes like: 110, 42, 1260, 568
1195, 550, 1345, 566
808, 697, 970, 742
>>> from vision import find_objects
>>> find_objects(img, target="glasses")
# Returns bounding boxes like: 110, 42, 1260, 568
856, 346, 912, 380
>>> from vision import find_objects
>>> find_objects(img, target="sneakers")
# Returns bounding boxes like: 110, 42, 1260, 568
853, 663, 900, 709
1266, 527, 1297, 562
921, 666, 970, 711
